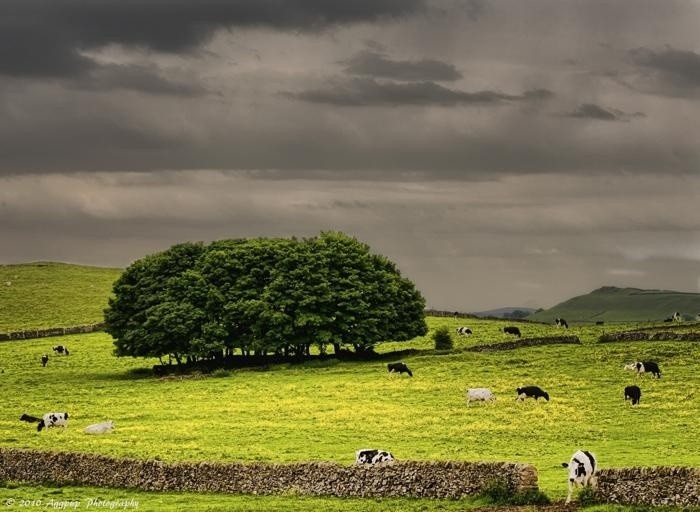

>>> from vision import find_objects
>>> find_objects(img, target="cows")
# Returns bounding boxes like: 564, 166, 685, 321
671, 311, 681, 322
624, 385, 641, 405
554, 317, 569, 330
387, 362, 413, 379
466, 388, 496, 407
355, 449, 395, 467
516, 386, 549, 404
623, 360, 662, 380
19, 412, 70, 432
53, 345, 69, 356
561, 450, 598, 506
83, 420, 115, 435
503, 326, 522, 339
41, 355, 49, 367
457, 326, 472, 338
